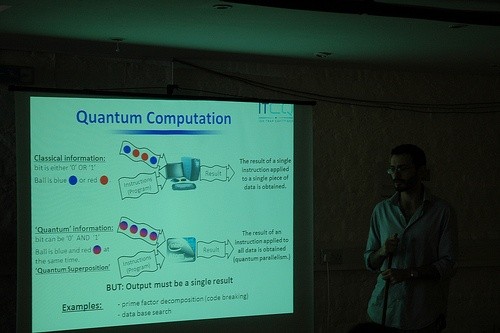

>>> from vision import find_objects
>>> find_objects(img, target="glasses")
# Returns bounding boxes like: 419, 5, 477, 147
386, 164, 418, 176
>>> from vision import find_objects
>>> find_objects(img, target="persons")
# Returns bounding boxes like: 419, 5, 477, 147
361, 143, 459, 333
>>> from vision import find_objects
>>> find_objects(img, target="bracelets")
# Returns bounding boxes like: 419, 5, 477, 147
407, 269, 414, 280
